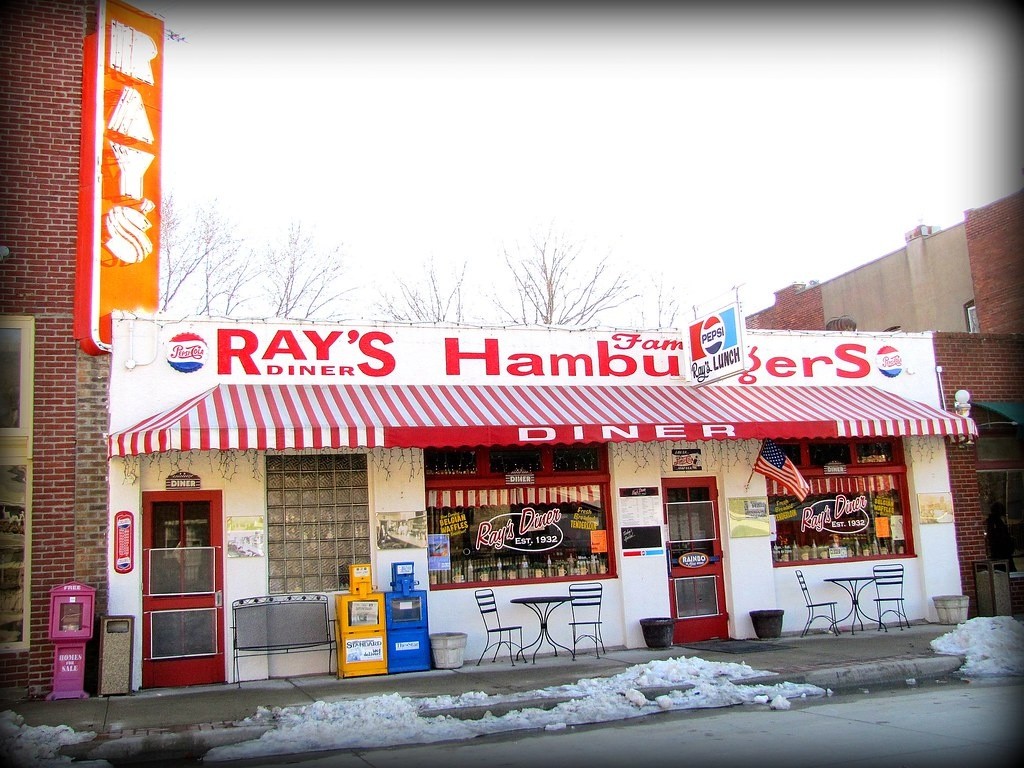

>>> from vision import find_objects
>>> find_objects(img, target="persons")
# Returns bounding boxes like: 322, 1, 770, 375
986, 502, 1018, 572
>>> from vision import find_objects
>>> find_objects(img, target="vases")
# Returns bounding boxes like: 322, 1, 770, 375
750, 609, 784, 639
639, 617, 675, 648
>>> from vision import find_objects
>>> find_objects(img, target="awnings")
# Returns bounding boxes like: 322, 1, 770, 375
970, 401, 1024, 425
107, 385, 980, 463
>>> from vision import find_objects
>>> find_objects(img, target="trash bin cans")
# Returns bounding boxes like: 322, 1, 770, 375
85, 612, 135, 695
970, 558, 1012, 617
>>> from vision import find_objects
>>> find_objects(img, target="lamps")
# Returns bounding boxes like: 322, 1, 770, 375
954, 389, 971, 416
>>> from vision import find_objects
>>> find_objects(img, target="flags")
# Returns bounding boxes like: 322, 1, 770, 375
753, 437, 810, 502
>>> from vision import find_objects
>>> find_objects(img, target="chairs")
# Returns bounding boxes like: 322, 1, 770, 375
474, 588, 527, 666
794, 570, 841, 636
873, 563, 910, 631
567, 582, 605, 661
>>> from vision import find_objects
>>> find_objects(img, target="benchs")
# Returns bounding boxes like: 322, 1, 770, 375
228, 593, 339, 688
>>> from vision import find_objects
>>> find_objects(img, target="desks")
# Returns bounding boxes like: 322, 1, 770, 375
509, 595, 575, 664
824, 576, 887, 635
